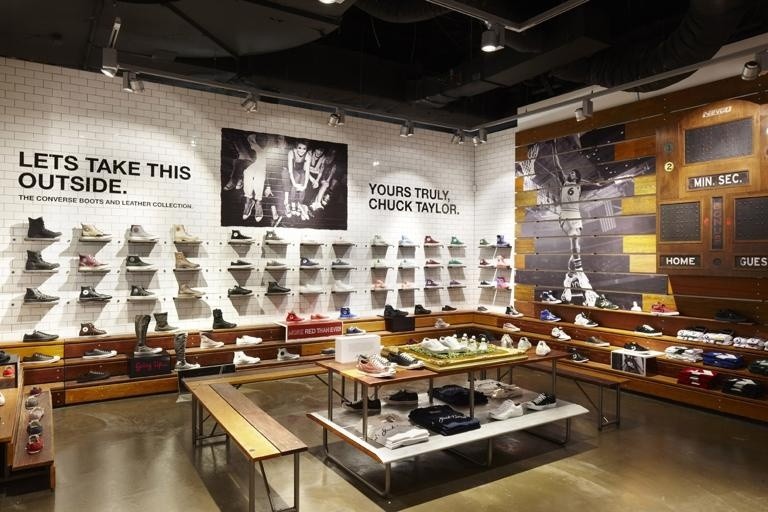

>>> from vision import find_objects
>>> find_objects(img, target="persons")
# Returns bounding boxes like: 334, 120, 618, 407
224, 135, 343, 224
546, 138, 616, 289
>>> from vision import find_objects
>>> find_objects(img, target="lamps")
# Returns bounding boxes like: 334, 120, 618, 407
482, 29, 496, 52
130, 78, 145, 93
327, 113, 339, 127
240, 98, 256, 112
740, 61, 762, 82
123, 71, 137, 92
472, 135, 480, 146
100, 64, 121, 78
575, 108, 586, 122
451, 136, 460, 144
400, 126, 409, 137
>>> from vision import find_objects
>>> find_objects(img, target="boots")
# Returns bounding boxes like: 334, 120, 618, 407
175, 331, 201, 370
212, 309, 237, 330
495, 277, 509, 289
26, 249, 60, 271
133, 315, 163, 355
175, 251, 200, 269
28, 217, 62, 238
152, 312, 179, 331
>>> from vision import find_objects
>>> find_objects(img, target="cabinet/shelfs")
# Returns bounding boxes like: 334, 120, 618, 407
306, 344, 591, 498
65, 321, 302, 405
0, 336, 64, 409
472, 309, 768, 424
301, 306, 474, 362
0, 353, 56, 493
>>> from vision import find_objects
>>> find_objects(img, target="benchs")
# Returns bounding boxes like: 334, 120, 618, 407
180, 378, 308, 512
182, 364, 358, 435
521, 360, 631, 432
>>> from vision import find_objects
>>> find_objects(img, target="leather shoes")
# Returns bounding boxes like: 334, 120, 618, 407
24, 287, 61, 303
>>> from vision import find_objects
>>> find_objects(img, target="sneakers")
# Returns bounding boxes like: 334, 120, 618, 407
235, 334, 263, 347
479, 280, 491, 287
449, 279, 462, 285
434, 319, 451, 328
25, 434, 44, 454
503, 323, 520, 331
131, 285, 156, 299
30, 386, 43, 396
424, 259, 441, 267
382, 389, 418, 405
497, 235, 509, 247
713, 308, 749, 323
178, 281, 205, 298
356, 353, 396, 378
439, 334, 467, 353
488, 399, 523, 420
626, 342, 650, 354
319, 346, 336, 356
451, 236, 465, 246
341, 397, 381, 413
650, 303, 680, 316
594, 294, 620, 310
505, 306, 524, 317
311, 313, 330, 321
79, 285, 111, 300
448, 260, 464, 267
284, 204, 292, 218
76, 369, 111, 383
332, 279, 357, 293
26, 418, 43, 435
425, 278, 440, 288
229, 230, 253, 242
340, 307, 359, 318
22, 329, 60, 343
345, 325, 366, 335
127, 224, 160, 243
276, 347, 300, 361
541, 290, 563, 304
199, 335, 225, 349
27, 407, 45, 421
586, 336, 610, 347
81, 347, 118, 360
271, 206, 279, 220
420, 336, 448, 354
264, 230, 292, 245
479, 239, 495, 247
265, 260, 289, 269
23, 352, 61, 364
500, 334, 514, 349
80, 223, 112, 237
267, 280, 291, 294
227, 284, 253, 298
633, 325, 663, 337
535, 340, 549, 356
233, 350, 261, 366
478, 258, 493, 268
384, 305, 409, 318
332, 258, 351, 268
254, 202, 264, 223
286, 311, 306, 323
576, 274, 593, 289
77, 253, 109, 271
125, 255, 158, 271
24, 394, 39, 410
424, 236, 442, 246
224, 182, 235, 190
291, 203, 325, 221
387, 351, 424, 370
236, 179, 244, 189
496, 255, 511, 267
573, 311, 599, 327
539, 309, 562, 322
477, 306, 489, 313
568, 349, 590, 363
563, 273, 575, 289
551, 327, 571, 341
173, 223, 204, 244
414, 304, 431, 315
525, 391, 557, 411
231, 259, 254, 269
78, 323, 107, 337
517, 336, 532, 353
441, 305, 457, 312
2, 366, 15, 377
300, 257, 319, 266
242, 198, 255, 220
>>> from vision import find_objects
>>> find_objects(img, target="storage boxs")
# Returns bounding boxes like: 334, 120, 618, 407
335, 334, 382, 363
